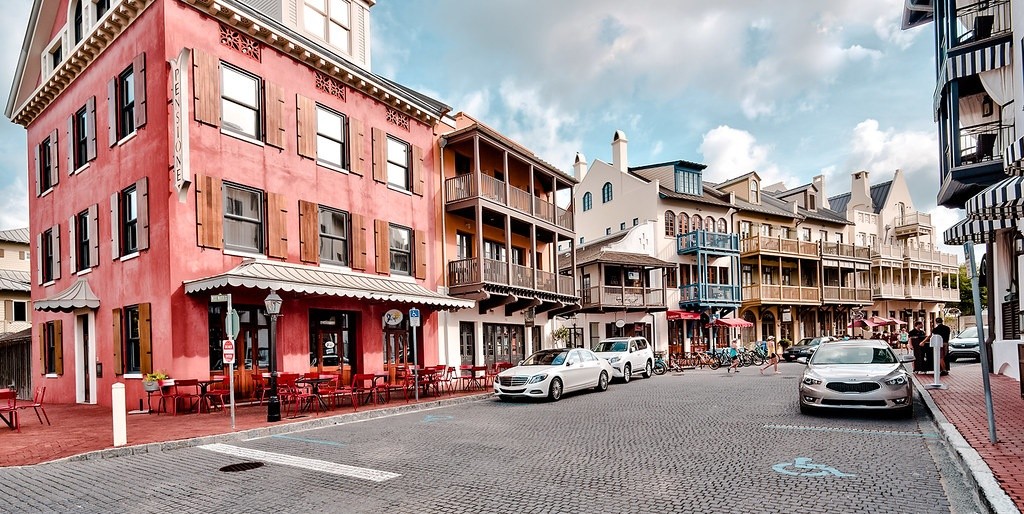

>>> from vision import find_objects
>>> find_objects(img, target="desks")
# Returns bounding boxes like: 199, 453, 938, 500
295, 376, 331, 413
411, 369, 433, 398
191, 379, 223, 414
361, 375, 388, 404
459, 368, 485, 391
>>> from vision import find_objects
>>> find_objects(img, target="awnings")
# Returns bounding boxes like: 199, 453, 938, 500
716, 318, 754, 328
666, 311, 700, 320
33, 278, 100, 313
943, 135, 1024, 245
182, 259, 476, 313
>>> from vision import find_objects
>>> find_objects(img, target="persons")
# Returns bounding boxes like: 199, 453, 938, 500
871, 330, 901, 348
909, 318, 950, 376
899, 328, 909, 355
728, 339, 739, 372
760, 336, 781, 374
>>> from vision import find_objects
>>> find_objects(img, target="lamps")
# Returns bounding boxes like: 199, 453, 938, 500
1013, 230, 1024, 256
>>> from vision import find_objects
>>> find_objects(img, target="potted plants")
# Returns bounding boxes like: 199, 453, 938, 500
143, 373, 168, 390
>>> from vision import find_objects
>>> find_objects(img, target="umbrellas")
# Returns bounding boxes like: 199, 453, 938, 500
848, 316, 908, 338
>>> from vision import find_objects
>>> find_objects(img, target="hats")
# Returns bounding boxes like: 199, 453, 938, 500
768, 336, 775, 339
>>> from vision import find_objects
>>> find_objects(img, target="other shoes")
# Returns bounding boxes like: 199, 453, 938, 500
774, 370, 782, 374
735, 370, 740, 373
727, 367, 730, 373
760, 368, 764, 374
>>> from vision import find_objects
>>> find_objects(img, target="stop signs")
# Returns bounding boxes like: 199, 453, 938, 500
224, 341, 234, 362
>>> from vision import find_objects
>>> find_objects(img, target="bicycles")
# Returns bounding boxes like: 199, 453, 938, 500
652, 356, 667, 376
693, 347, 781, 370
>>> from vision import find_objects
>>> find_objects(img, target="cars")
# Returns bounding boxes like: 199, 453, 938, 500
797, 338, 917, 419
589, 336, 655, 385
782, 336, 838, 363
948, 326, 989, 362
494, 348, 614, 402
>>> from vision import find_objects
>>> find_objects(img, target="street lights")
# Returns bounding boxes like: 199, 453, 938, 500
263, 290, 283, 423
570, 314, 580, 348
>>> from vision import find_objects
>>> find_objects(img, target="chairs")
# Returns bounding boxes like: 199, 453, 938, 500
156, 363, 512, 419
961, 134, 998, 164
0, 385, 51, 433
957, 14, 995, 45
448, 259, 499, 283
